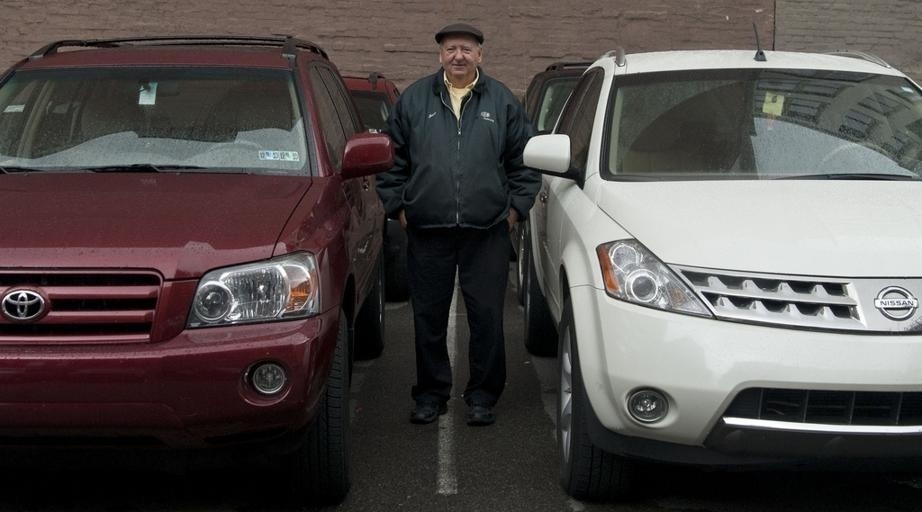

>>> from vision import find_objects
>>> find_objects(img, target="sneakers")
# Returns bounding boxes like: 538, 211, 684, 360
408, 401, 449, 426
465, 403, 498, 427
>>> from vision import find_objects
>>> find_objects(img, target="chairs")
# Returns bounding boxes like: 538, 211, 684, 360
44, 80, 293, 151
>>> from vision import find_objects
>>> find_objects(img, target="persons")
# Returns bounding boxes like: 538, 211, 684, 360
373, 22, 545, 428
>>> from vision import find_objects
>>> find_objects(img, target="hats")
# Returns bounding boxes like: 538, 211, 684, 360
433, 22, 485, 46
